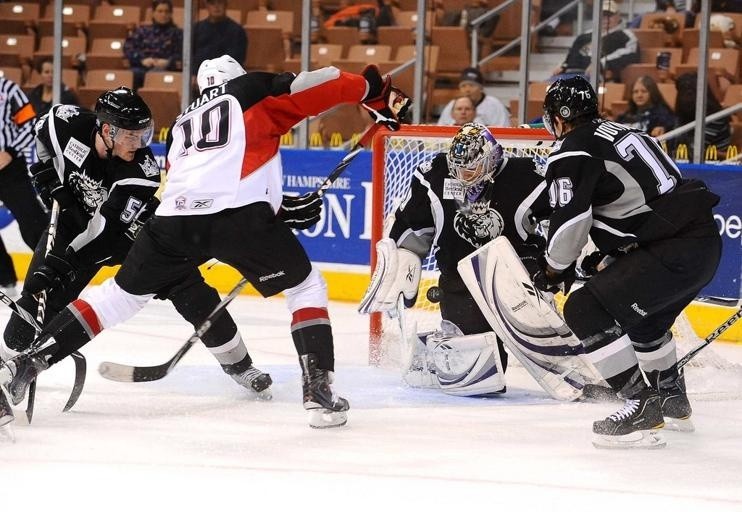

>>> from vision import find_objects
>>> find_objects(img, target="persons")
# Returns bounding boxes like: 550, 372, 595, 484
1, 85, 269, 406
380, 123, 562, 394
176, 4, 248, 77
521, 3, 639, 128
330, 2, 393, 44
536, 3, 580, 34
529, 73, 724, 437
27, 56, 80, 117
436, 2, 500, 60
626, 0, 698, 28
0, 55, 412, 413
652, 74, 731, 159
449, 97, 484, 127
436, 68, 513, 126
0, 70, 48, 290
612, 74, 674, 137
123, 4, 183, 88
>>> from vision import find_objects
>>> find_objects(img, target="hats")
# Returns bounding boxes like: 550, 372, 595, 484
458, 67, 483, 88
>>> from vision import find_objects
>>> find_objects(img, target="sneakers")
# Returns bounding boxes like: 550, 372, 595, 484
594, 389, 665, 435
660, 368, 692, 419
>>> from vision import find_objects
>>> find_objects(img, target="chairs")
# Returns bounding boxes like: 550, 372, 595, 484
1, 0, 742, 164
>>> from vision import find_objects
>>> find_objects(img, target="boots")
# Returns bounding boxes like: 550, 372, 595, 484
298, 353, 349, 409
233, 365, 273, 391
5, 330, 57, 406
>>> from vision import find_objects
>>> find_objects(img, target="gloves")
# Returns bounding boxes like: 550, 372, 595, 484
580, 252, 611, 277
358, 63, 413, 132
29, 159, 76, 216
278, 191, 322, 230
26, 241, 85, 294
532, 251, 577, 295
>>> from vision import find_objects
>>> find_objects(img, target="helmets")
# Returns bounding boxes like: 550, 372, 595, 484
94, 85, 154, 148
445, 121, 504, 204
196, 54, 247, 97
541, 73, 598, 140
